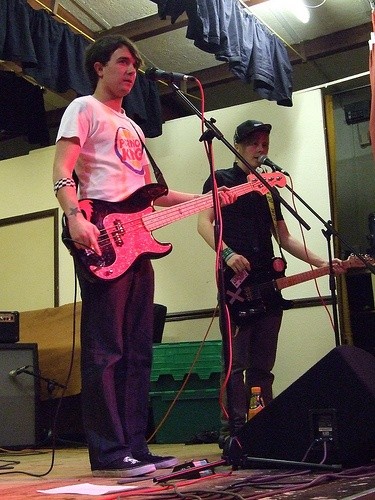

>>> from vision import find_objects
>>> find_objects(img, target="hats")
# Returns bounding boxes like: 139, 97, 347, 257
233, 120, 272, 144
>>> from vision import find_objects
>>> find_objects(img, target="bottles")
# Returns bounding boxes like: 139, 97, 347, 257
248, 387, 264, 421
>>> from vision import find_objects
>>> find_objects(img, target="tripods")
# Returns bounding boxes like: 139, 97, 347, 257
154, 73, 341, 483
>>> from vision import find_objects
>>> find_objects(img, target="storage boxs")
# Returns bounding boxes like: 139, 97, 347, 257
151, 340, 223, 442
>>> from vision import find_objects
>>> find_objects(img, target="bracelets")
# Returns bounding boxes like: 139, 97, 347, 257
53, 178, 76, 195
224, 252, 236, 262
222, 247, 234, 259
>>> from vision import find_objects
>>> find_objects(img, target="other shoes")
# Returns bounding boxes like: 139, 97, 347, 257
133, 447, 177, 468
92, 455, 156, 477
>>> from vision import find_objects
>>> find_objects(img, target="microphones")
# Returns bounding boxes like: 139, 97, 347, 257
8, 365, 29, 376
257, 155, 290, 176
146, 67, 196, 82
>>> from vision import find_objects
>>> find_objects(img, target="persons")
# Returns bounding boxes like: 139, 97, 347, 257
51, 35, 238, 477
197, 119, 347, 450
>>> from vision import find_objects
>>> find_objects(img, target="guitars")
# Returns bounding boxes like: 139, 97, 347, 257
62, 166, 287, 282
217, 252, 375, 325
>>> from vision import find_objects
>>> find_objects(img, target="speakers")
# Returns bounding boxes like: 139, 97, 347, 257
0, 342, 41, 451
223, 344, 375, 466
